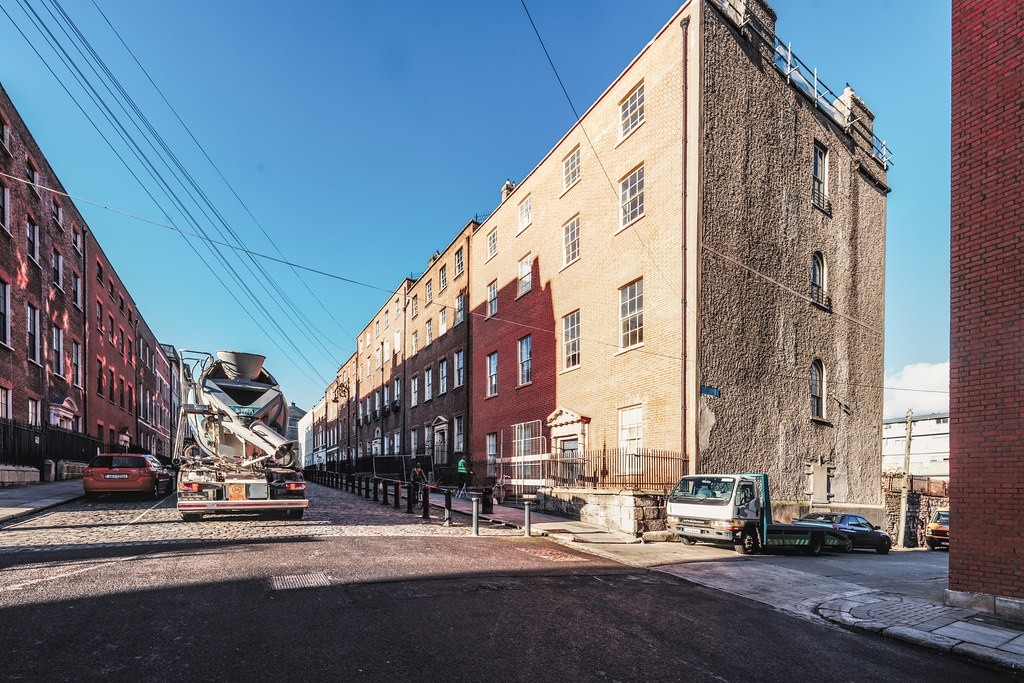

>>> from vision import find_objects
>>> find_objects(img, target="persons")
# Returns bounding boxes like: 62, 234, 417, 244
410, 463, 428, 504
458, 456, 473, 487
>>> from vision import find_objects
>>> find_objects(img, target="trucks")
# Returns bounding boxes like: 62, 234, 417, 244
666, 474, 848, 557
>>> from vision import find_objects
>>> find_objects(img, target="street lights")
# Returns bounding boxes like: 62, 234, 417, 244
332, 385, 350, 474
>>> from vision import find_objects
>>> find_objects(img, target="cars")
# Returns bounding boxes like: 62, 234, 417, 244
790, 512, 892, 555
82, 453, 172, 500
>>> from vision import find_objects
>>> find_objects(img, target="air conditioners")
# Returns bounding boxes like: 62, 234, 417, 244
357, 417, 363, 428
373, 410, 380, 422
391, 400, 400, 412
380, 405, 389, 417
364, 414, 371, 425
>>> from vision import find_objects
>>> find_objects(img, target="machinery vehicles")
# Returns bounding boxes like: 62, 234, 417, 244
172, 348, 308, 519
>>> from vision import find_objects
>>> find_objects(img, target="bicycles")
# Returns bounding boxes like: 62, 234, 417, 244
412, 480, 426, 510
492, 474, 511, 503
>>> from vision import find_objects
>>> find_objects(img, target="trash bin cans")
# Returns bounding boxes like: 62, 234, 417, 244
481, 487, 494, 514
44, 459, 56, 484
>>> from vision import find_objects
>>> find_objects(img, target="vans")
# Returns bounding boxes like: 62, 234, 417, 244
925, 507, 949, 550
